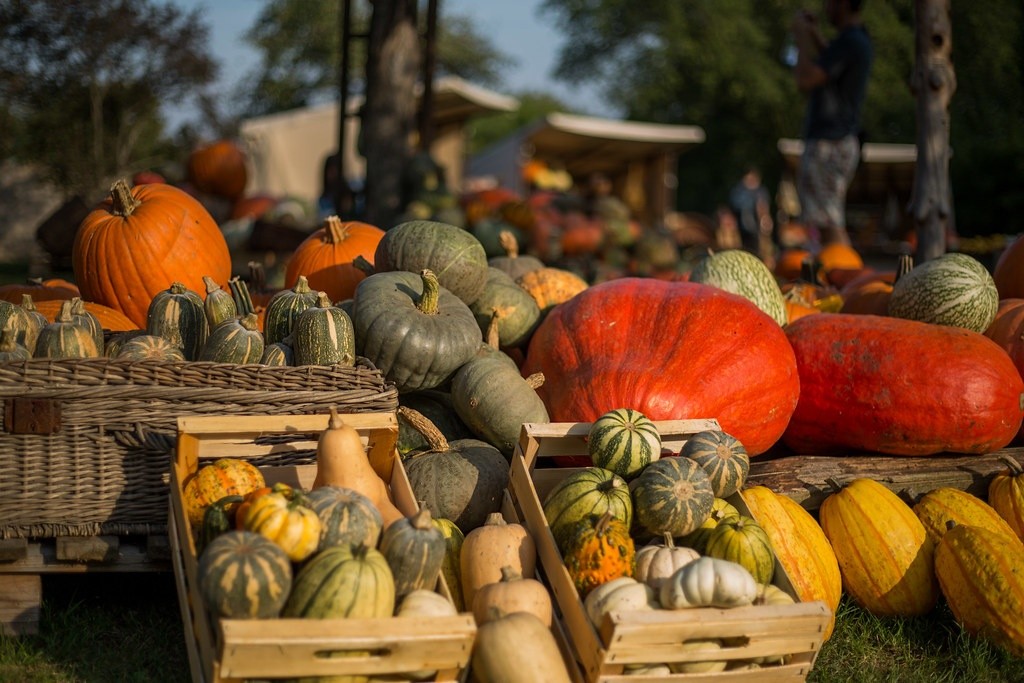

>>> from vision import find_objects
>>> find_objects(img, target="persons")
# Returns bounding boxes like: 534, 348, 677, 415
778, 1, 880, 285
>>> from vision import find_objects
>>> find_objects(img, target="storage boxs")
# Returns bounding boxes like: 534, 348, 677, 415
499, 415, 831, 683
171, 406, 480, 683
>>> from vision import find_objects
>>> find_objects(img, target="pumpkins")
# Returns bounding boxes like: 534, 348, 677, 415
0, 112, 1021, 683
